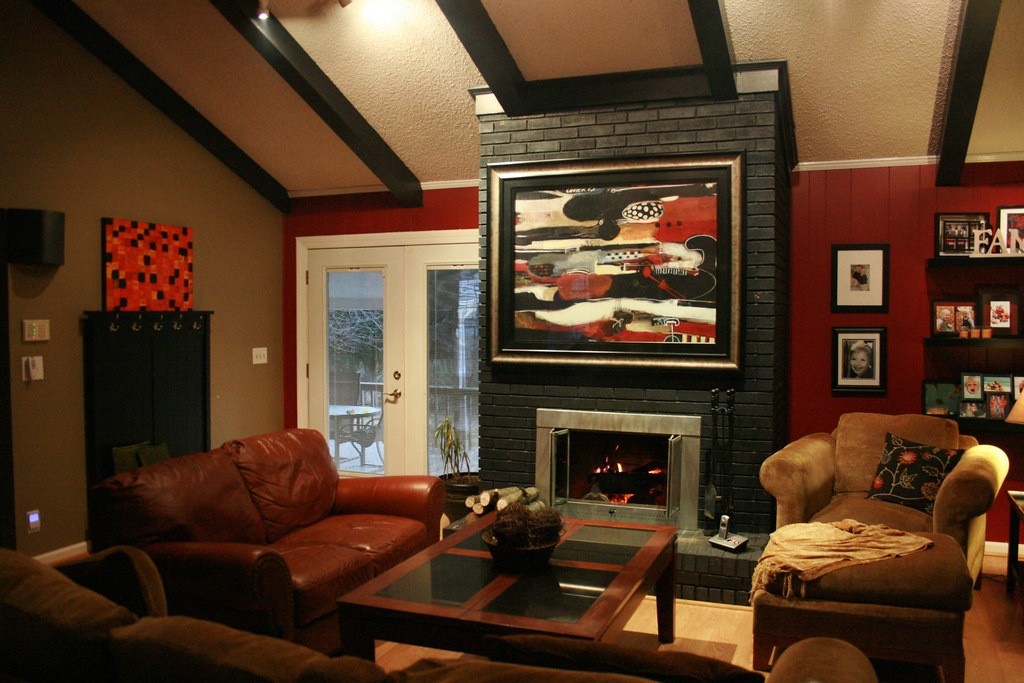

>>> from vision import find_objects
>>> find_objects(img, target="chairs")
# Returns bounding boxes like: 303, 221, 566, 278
338, 405, 385, 469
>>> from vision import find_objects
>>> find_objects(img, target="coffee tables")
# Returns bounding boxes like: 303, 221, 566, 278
335, 517, 679, 661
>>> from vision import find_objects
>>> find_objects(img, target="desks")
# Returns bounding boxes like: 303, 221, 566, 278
1004, 489, 1024, 595
330, 405, 380, 467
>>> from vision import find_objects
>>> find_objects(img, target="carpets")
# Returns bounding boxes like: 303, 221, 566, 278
372, 632, 738, 667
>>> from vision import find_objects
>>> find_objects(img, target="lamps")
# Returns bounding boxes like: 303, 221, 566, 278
3, 207, 67, 267
1005, 388, 1024, 425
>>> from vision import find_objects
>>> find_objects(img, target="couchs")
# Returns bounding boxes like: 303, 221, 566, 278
87, 428, 444, 656
758, 412, 1011, 598
1, 548, 877, 683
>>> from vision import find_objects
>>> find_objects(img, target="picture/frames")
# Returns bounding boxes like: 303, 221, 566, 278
977, 283, 1024, 340
984, 374, 1014, 392
997, 206, 1024, 254
961, 372, 983, 400
959, 401, 984, 420
934, 302, 977, 335
484, 150, 747, 375
934, 213, 990, 256
985, 394, 1015, 422
830, 243, 889, 314
831, 327, 888, 396
921, 378, 962, 420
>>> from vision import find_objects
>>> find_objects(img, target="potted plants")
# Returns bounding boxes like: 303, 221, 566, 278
483, 506, 563, 559
437, 419, 481, 505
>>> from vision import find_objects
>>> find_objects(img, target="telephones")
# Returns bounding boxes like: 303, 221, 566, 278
709, 514, 750, 553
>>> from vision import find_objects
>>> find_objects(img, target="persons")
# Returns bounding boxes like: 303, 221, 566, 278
847, 341, 875, 379
850, 265, 870, 290
936, 310, 974, 332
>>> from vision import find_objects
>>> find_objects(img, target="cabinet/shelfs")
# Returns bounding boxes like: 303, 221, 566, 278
924, 254, 1024, 432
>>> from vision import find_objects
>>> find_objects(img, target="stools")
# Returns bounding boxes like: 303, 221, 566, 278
753, 529, 976, 683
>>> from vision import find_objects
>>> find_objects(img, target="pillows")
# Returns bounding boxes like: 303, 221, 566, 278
866, 432, 965, 514
